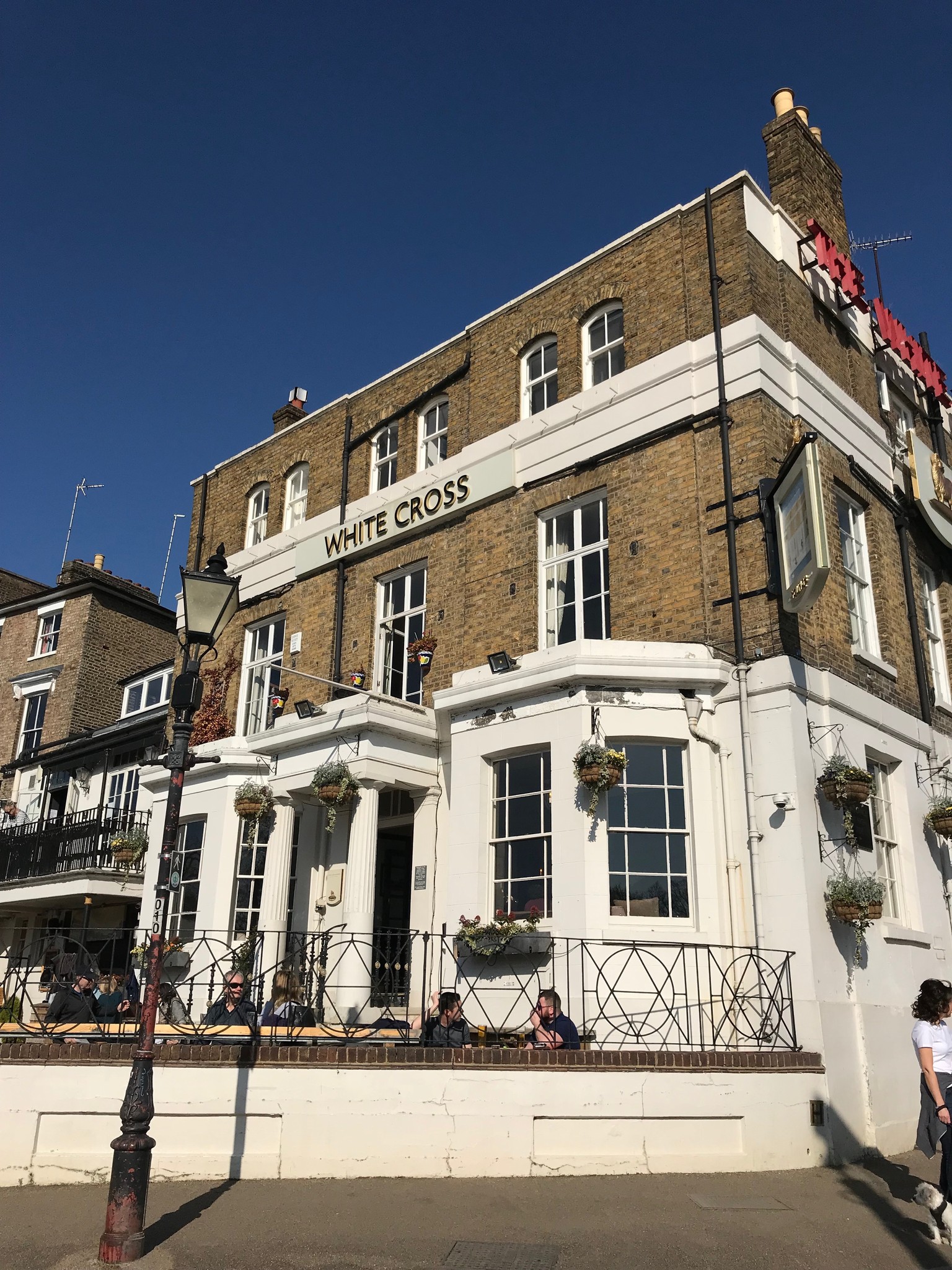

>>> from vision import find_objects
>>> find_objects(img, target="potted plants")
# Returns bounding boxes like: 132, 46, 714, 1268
311, 760, 360, 833
271, 690, 289, 710
827, 872, 885, 962
349, 664, 366, 689
234, 777, 274, 849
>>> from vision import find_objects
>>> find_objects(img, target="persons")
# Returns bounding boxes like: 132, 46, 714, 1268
910, 977, 952, 1205
411, 991, 473, 1048
157, 983, 186, 1044
258, 970, 314, 1046
43, 964, 129, 1043
197, 971, 261, 1045
523, 990, 581, 1050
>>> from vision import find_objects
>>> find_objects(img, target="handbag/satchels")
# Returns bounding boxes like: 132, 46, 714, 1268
289, 1006, 316, 1027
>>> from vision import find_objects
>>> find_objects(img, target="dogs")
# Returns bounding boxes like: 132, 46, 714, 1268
912, 1181, 952, 1247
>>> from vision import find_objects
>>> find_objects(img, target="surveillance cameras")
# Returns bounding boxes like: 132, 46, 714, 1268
772, 796, 789, 808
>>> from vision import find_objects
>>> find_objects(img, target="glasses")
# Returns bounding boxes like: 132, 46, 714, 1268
230, 983, 243, 989
537, 1005, 553, 1011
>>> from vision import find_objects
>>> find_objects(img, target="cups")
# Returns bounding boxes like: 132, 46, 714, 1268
247, 1012, 255, 1026
516, 1026, 525, 1049
478, 1025, 487, 1048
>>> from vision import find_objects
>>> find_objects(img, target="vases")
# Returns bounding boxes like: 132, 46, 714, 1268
578, 763, 621, 785
822, 780, 869, 802
933, 817, 952, 835
144, 951, 190, 968
455, 931, 551, 953
417, 651, 432, 670
113, 848, 144, 862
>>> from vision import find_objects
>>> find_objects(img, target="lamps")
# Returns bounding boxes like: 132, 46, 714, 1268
294, 699, 322, 719
145, 745, 161, 765
487, 650, 516, 674
76, 764, 93, 794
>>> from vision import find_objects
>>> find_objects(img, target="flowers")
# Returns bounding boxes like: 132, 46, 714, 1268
459, 904, 542, 954
129, 936, 184, 969
108, 826, 148, 860
821, 755, 877, 845
573, 744, 630, 813
406, 631, 437, 663
925, 796, 952, 820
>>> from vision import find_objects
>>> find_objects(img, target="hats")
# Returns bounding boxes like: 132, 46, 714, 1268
75, 965, 100, 979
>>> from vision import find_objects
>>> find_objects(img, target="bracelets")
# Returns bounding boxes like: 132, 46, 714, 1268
936, 1104, 947, 1112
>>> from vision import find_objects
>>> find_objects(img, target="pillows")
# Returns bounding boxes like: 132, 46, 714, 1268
614, 896, 659, 917
